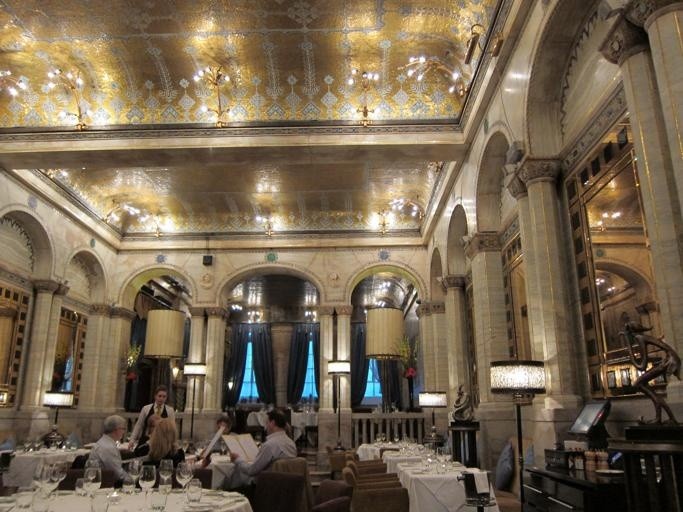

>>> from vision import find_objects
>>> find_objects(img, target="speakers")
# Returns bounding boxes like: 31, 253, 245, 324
203, 256, 212, 264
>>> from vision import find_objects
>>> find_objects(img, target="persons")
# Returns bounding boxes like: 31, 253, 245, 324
134, 414, 165, 459
82, 414, 135, 487
228, 408, 297, 478
128, 384, 174, 450
130, 416, 185, 479
200, 415, 241, 453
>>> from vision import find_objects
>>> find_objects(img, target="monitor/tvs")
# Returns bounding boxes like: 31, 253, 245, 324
567, 399, 612, 445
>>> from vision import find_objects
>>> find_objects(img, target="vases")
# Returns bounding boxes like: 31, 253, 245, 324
407, 375, 422, 412
124, 380, 133, 412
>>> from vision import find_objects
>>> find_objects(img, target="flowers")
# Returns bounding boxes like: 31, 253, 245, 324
119, 341, 142, 380
395, 335, 417, 378
53, 344, 67, 385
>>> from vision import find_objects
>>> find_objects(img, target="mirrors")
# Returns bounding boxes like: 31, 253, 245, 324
52, 307, 87, 409
565, 108, 683, 400
467, 284, 479, 390
502, 231, 532, 361
0, 282, 32, 408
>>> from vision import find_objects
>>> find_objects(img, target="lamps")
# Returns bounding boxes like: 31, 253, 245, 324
419, 392, 448, 439
102, 200, 149, 239
490, 361, 546, 512
389, 200, 426, 221
194, 65, 230, 128
43, 391, 74, 448
46, 68, 89, 130
254, 215, 282, 239
349, 68, 380, 128
328, 361, 351, 450
1, 69, 27, 99
143, 308, 186, 384
396, 55, 465, 97
184, 363, 206, 438
368, 209, 395, 238
366, 307, 404, 412
138, 207, 175, 239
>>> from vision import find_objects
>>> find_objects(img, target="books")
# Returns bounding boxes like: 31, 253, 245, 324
221, 432, 260, 461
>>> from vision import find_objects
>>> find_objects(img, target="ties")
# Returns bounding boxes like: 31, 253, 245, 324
156, 404, 162, 415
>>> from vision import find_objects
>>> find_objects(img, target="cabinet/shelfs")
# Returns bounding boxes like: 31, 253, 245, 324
523, 467, 668, 512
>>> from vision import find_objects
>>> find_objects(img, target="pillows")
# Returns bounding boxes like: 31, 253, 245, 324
496, 440, 514, 490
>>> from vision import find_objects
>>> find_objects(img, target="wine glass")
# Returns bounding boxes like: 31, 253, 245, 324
21, 434, 80, 453
16, 457, 204, 512
376, 431, 456, 477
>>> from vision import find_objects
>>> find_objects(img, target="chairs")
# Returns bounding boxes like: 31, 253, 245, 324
252, 446, 409, 512
495, 439, 534, 512
